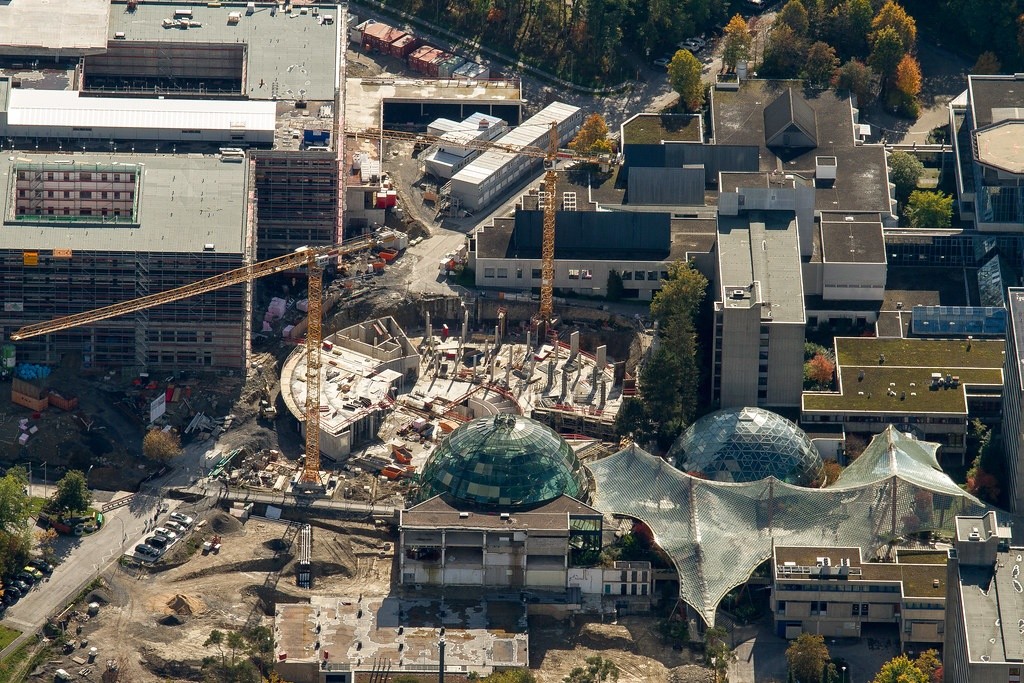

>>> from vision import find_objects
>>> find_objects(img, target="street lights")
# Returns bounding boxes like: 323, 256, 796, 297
86, 465, 93, 491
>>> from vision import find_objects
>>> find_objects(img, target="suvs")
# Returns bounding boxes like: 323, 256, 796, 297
144, 536, 167, 549
154, 527, 176, 541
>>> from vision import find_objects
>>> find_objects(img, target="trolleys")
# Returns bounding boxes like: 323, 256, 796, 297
464, 349, 485, 363
411, 424, 434, 440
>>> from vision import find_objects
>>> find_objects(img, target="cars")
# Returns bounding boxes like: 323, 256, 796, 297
12, 572, 35, 584
654, 58, 671, 68
0, 580, 30, 612
163, 520, 186, 534
28, 557, 54, 574
686, 37, 706, 47
169, 512, 193, 527
135, 544, 160, 557
677, 42, 699, 53
23, 565, 43, 579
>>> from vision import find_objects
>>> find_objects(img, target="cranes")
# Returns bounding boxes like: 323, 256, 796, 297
288, 116, 626, 337
8, 229, 402, 493
